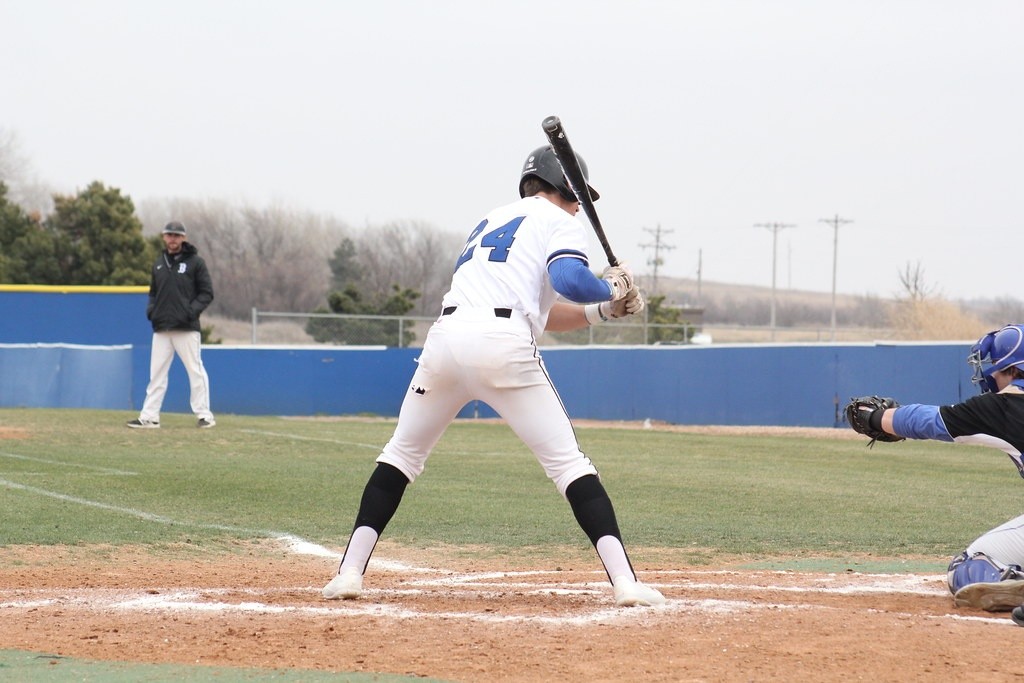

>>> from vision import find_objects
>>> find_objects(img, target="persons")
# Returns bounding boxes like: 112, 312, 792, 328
847, 324, 1024, 625
126, 222, 216, 429
321, 147, 667, 603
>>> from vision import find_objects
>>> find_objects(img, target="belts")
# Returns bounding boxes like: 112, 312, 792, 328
443, 306, 512, 318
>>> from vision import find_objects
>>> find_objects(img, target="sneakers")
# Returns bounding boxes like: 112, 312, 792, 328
614, 576, 666, 605
322, 571, 363, 600
197, 418, 216, 428
126, 418, 160, 429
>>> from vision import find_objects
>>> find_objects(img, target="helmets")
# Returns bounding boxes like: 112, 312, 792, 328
163, 220, 186, 236
990, 323, 1024, 371
519, 145, 600, 205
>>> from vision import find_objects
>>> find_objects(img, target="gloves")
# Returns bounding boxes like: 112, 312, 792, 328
610, 285, 645, 318
602, 261, 633, 302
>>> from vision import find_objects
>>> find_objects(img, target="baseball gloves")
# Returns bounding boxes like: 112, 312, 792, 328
842, 394, 907, 450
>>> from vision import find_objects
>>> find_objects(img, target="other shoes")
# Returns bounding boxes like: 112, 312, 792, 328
1011, 605, 1024, 627
953, 575, 1024, 611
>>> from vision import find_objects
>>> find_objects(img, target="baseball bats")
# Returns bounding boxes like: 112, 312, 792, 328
542, 115, 634, 315
